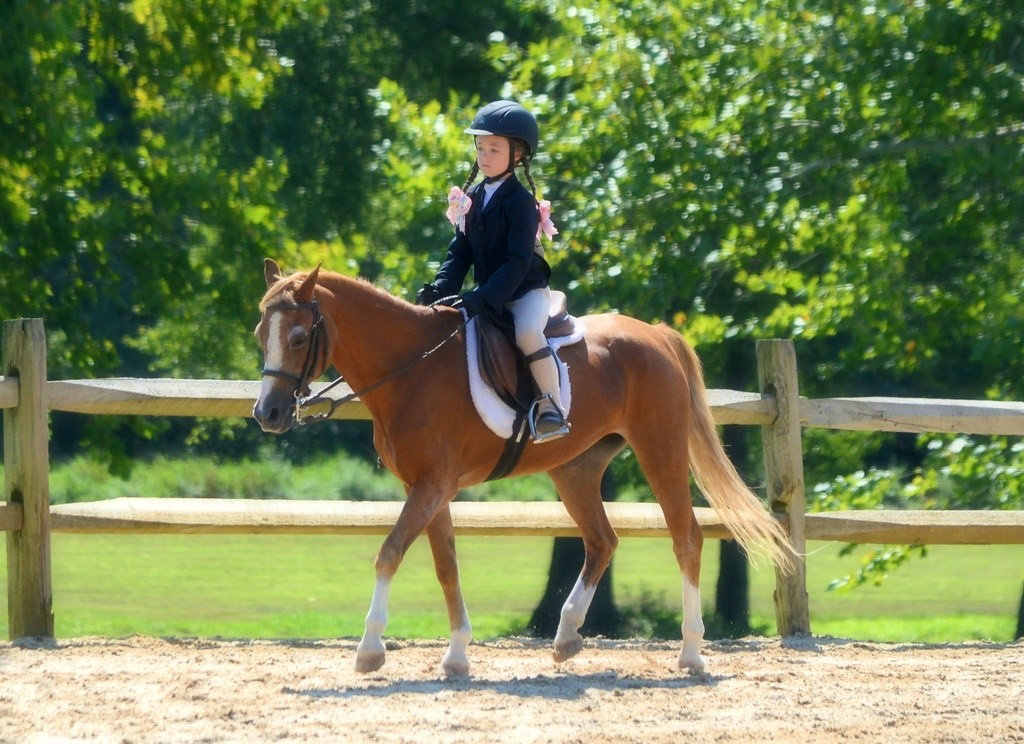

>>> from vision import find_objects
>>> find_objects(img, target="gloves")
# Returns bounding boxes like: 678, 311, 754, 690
451, 291, 487, 318
415, 282, 441, 307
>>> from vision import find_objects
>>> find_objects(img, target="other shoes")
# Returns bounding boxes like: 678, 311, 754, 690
534, 406, 567, 436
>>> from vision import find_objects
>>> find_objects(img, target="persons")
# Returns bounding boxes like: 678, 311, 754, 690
412, 99, 571, 436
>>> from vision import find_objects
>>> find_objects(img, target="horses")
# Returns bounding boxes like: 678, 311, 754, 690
251, 253, 804, 677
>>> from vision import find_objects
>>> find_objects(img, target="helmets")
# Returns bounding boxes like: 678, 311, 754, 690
463, 100, 539, 158
